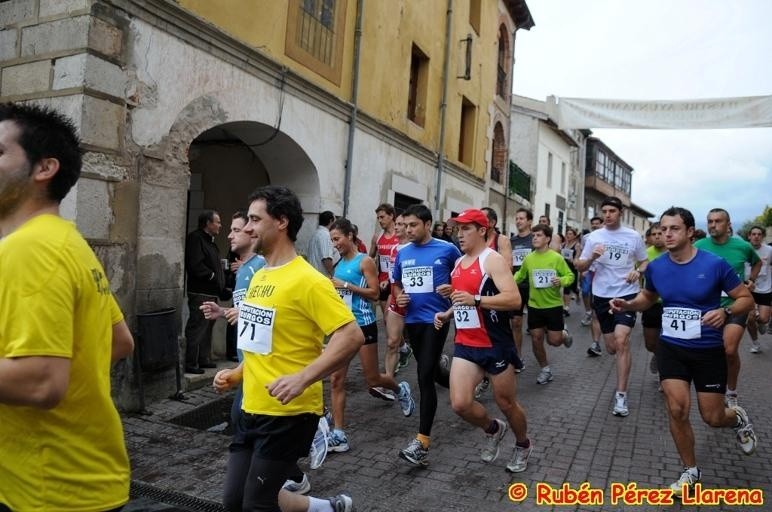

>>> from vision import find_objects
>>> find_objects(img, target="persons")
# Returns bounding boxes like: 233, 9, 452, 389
213, 185, 367, 511
199, 210, 330, 497
310, 198, 771, 474
0, 101, 134, 511
185, 209, 223, 374
223, 242, 241, 362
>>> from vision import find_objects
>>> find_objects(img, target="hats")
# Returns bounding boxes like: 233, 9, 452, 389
600, 197, 622, 209
446, 209, 490, 231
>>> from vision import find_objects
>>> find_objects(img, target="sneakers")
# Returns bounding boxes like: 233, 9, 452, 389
199, 362, 217, 368
650, 354, 658, 374
281, 474, 311, 496
479, 419, 507, 464
328, 433, 349, 453
515, 359, 525, 373
750, 341, 760, 352
563, 306, 570, 317
506, 438, 534, 474
537, 368, 553, 384
571, 295, 576, 300
397, 381, 416, 417
587, 343, 602, 356
323, 406, 333, 426
325, 494, 352, 512
612, 393, 629, 416
733, 406, 756, 455
576, 295, 581, 306
671, 467, 701, 498
756, 314, 766, 334
727, 390, 738, 409
473, 377, 489, 399
440, 355, 449, 368
369, 387, 395, 401
581, 312, 593, 326
186, 367, 205, 375
565, 329, 573, 347
398, 345, 413, 367
658, 377, 664, 392
399, 439, 429, 466
228, 356, 240, 362
308, 417, 329, 470
